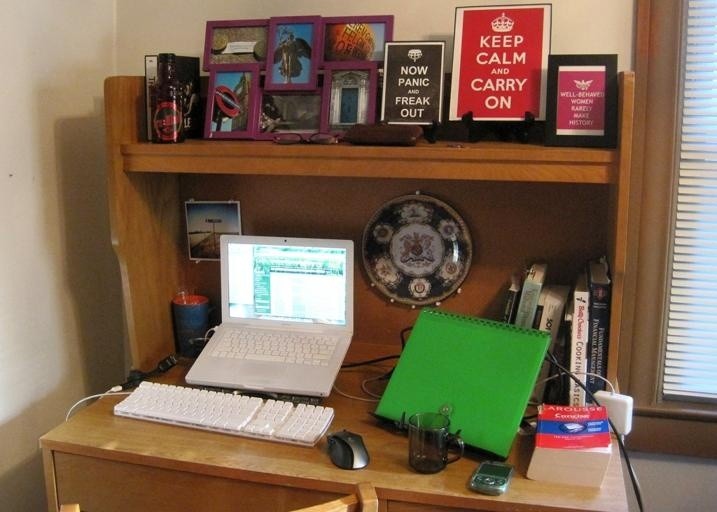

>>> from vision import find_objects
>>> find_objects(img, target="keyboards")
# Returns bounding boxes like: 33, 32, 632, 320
113, 379, 335, 449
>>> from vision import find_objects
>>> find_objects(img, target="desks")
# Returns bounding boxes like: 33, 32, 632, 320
35, 351, 634, 511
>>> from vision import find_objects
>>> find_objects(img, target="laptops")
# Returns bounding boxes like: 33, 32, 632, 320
184, 235, 355, 398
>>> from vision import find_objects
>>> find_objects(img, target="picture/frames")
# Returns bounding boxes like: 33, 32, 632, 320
263, 14, 321, 90
253, 87, 330, 140
202, 63, 258, 139
201, 19, 268, 72
545, 53, 618, 149
309, 13, 393, 69
319, 60, 377, 135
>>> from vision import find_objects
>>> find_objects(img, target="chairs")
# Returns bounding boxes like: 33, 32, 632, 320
55, 478, 379, 512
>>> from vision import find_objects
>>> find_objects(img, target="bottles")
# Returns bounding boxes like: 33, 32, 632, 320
149, 54, 184, 143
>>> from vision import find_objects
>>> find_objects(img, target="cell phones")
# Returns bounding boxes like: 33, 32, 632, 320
468, 460, 515, 496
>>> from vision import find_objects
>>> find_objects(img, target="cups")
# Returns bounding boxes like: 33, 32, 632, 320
408, 411, 465, 477
171, 295, 219, 356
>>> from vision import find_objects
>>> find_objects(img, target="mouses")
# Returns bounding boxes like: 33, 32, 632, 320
327, 429, 369, 470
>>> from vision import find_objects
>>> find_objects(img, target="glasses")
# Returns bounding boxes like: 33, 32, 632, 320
271, 132, 343, 146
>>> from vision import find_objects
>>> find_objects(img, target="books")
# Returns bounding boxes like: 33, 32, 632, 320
498, 257, 611, 409
522, 404, 614, 490
373, 308, 553, 458
